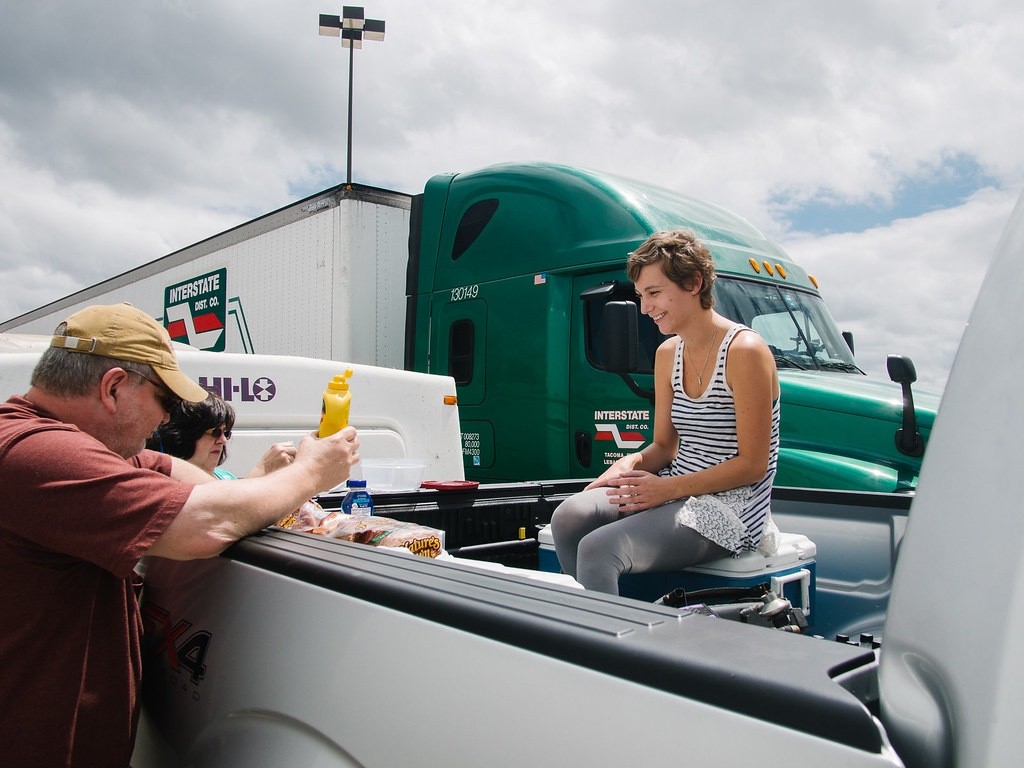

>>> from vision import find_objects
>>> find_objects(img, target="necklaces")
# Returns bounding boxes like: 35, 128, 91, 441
688, 324, 719, 386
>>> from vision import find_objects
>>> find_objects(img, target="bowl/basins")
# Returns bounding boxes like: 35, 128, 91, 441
360, 458, 427, 491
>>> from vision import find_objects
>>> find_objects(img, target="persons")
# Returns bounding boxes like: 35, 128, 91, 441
158, 392, 297, 478
0, 300, 360, 768
552, 231, 781, 596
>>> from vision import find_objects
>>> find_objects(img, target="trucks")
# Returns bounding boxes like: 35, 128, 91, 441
1, 158, 943, 510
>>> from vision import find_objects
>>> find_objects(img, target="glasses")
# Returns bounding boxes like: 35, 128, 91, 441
206, 428, 230, 438
99, 368, 184, 409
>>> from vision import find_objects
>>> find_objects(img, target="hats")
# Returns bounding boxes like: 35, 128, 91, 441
50, 302, 209, 403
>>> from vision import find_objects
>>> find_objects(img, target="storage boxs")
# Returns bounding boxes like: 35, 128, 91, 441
538, 524, 816, 639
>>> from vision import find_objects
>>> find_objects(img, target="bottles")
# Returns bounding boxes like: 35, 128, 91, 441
340, 480, 374, 517
317, 367, 353, 438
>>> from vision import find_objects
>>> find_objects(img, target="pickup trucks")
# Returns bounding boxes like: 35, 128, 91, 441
130, 175, 1024, 768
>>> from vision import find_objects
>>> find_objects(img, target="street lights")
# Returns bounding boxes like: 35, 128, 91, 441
316, 5, 386, 182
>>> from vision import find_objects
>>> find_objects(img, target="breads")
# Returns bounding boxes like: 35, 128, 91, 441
278, 502, 443, 559
